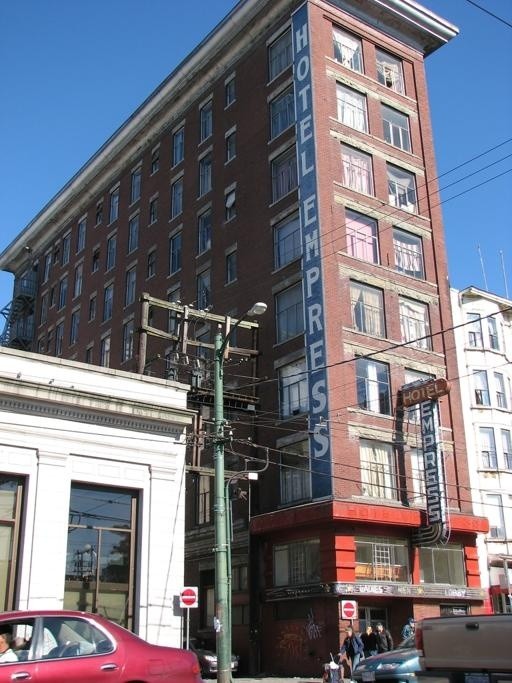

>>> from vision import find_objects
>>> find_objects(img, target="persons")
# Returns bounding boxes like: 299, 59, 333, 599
0, 632, 19, 663
338, 616, 418, 683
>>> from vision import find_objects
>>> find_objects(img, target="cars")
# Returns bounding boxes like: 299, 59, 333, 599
350, 632, 423, 683
0, 608, 206, 683
195, 645, 239, 676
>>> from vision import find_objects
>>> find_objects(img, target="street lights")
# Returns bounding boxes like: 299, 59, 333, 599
212, 301, 271, 683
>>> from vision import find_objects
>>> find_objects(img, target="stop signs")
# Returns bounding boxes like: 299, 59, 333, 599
340, 598, 359, 626
178, 586, 199, 607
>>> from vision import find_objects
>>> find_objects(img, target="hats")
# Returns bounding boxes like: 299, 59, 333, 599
344, 628, 352, 632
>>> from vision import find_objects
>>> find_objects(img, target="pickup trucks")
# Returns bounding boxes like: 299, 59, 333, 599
414, 613, 512, 682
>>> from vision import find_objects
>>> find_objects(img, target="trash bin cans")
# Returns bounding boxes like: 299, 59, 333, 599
325, 661, 340, 683
